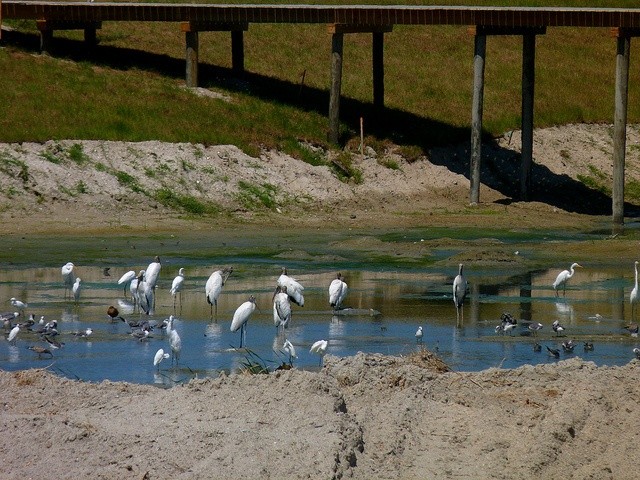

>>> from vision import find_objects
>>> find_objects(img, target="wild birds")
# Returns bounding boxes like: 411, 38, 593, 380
8, 297, 28, 317
6, 323, 22, 341
23, 313, 59, 337
167, 314, 182, 368
153, 348, 170, 373
143, 255, 162, 310
43, 336, 65, 349
328, 272, 343, 314
561, 340, 577, 352
629, 261, 640, 323
61, 262, 79, 301
543, 344, 560, 359
552, 320, 568, 337
25, 346, 53, 360
72, 277, 83, 308
276, 264, 305, 307
552, 263, 583, 298
169, 268, 189, 310
309, 339, 329, 368
0, 311, 20, 329
584, 342, 593, 351
118, 316, 168, 342
494, 312, 518, 337
453, 263, 467, 327
204, 266, 233, 323
136, 269, 154, 316
415, 326, 423, 345
335, 276, 352, 311
271, 286, 291, 345
230, 295, 260, 348
107, 306, 119, 322
117, 269, 136, 301
283, 338, 297, 363
69, 328, 93, 340
533, 343, 541, 352
528, 322, 544, 336
624, 325, 638, 335
